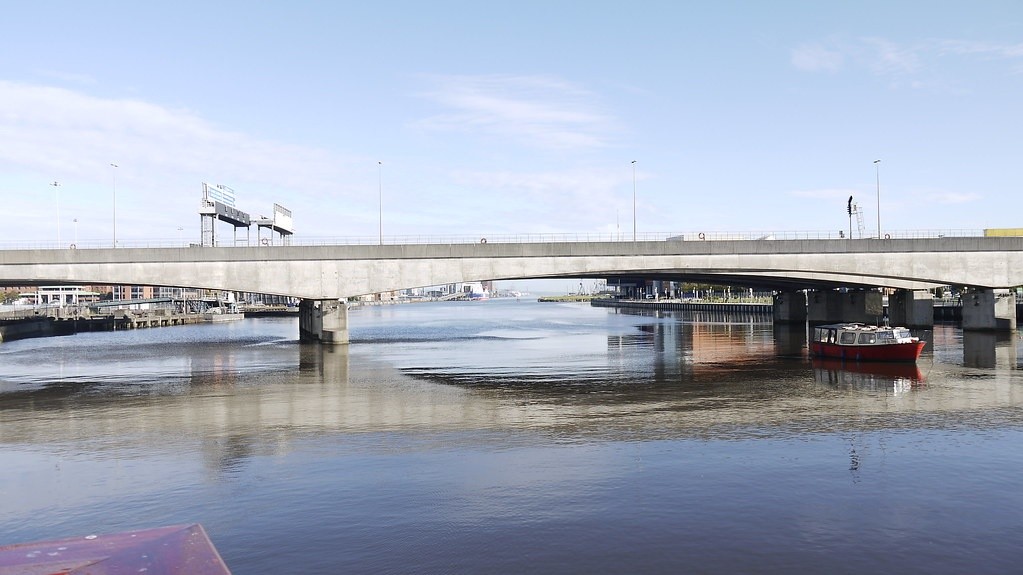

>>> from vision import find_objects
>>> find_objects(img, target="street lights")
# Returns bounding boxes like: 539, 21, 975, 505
873, 158, 882, 240
631, 159, 637, 242
110, 163, 118, 248
49, 181, 62, 249
378, 161, 382, 244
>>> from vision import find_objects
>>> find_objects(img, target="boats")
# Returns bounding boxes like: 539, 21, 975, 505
810, 354, 923, 381
812, 322, 927, 362
